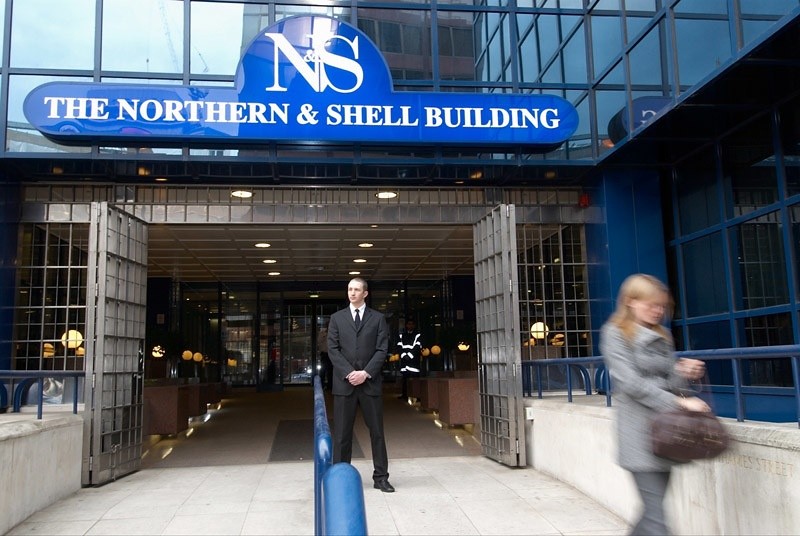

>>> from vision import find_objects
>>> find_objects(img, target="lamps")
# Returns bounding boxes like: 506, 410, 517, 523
389, 321, 565, 363
43, 329, 237, 368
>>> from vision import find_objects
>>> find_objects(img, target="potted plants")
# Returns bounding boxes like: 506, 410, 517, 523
142, 325, 226, 435
395, 296, 478, 425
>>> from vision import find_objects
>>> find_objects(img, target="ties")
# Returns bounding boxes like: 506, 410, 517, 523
354, 309, 361, 330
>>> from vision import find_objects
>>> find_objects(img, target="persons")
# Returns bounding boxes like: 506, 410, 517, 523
327, 277, 396, 492
598, 273, 710, 536
395, 318, 426, 404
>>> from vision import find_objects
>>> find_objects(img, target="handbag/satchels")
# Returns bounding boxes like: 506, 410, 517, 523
650, 408, 729, 464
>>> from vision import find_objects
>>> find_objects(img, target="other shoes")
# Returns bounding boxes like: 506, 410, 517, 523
373, 480, 395, 492
